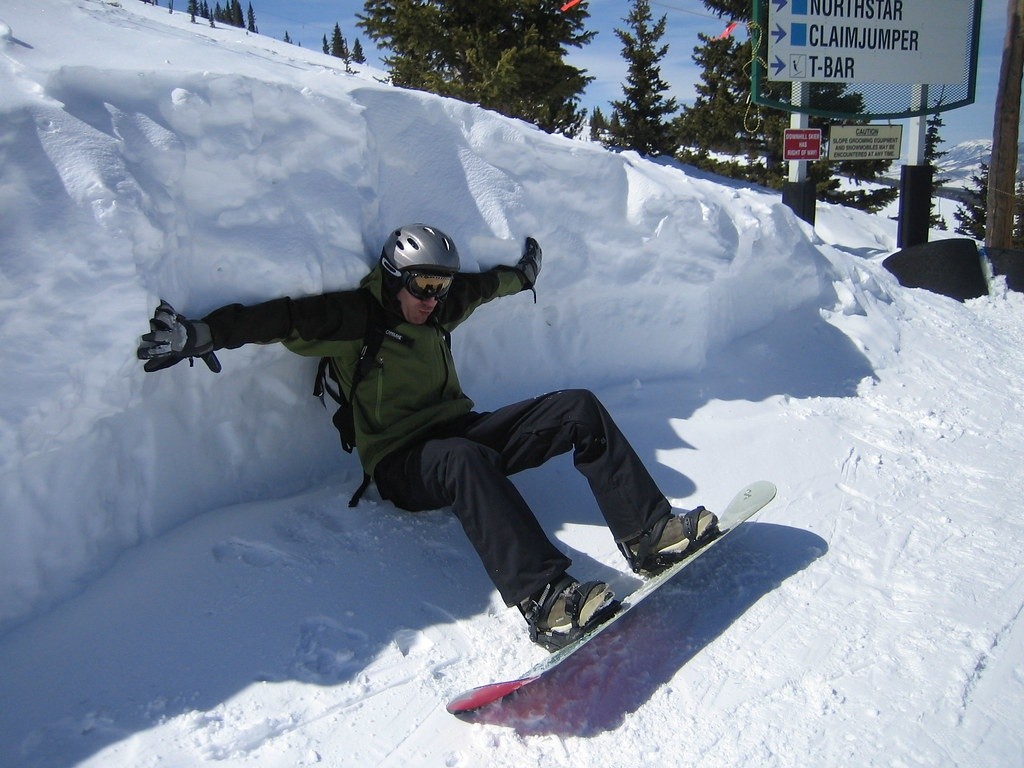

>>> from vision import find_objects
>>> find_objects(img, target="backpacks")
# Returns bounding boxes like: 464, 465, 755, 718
313, 294, 386, 454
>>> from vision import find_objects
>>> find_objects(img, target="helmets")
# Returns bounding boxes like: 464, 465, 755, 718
379, 222, 461, 304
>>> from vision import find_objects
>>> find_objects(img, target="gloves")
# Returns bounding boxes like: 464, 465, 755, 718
137, 299, 222, 373
515, 237, 542, 303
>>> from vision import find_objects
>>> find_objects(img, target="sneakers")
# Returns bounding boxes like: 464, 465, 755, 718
616, 506, 719, 580
516, 571, 622, 653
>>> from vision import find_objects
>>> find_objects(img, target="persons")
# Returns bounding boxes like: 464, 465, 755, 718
138, 224, 719, 644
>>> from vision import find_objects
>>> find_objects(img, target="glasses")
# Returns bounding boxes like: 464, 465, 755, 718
405, 272, 454, 304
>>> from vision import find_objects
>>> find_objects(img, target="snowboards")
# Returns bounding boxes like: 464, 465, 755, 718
440, 477, 777, 718
